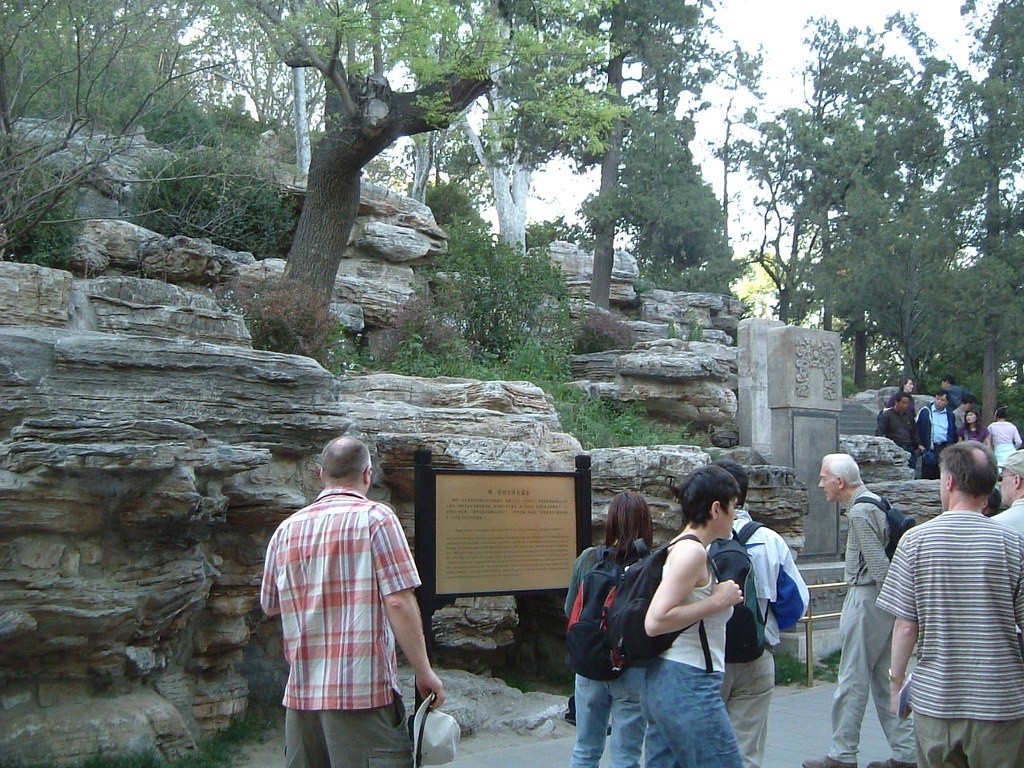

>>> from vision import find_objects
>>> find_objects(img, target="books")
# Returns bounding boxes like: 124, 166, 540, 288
897, 672, 914, 721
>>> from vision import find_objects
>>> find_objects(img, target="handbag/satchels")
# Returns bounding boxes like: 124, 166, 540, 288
915, 406, 934, 451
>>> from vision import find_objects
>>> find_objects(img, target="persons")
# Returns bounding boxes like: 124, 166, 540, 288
641, 464, 747, 768
801, 453, 923, 768
875, 373, 1024, 537
705, 464, 811, 768
260, 435, 446, 768
874, 440, 1024, 768
560, 490, 654, 768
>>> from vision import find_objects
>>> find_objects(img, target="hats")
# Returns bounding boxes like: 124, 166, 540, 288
998, 449, 1024, 479
414, 692, 461, 768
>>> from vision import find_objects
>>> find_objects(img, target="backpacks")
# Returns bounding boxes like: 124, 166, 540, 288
854, 494, 916, 564
601, 535, 721, 669
567, 544, 633, 681
703, 521, 769, 664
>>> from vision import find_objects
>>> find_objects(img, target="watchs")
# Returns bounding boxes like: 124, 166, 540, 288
887, 668, 907, 683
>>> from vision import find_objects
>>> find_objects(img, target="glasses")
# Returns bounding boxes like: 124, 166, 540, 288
997, 472, 1023, 482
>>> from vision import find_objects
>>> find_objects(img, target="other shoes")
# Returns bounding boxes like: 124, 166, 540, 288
802, 756, 857, 768
868, 758, 917, 768
